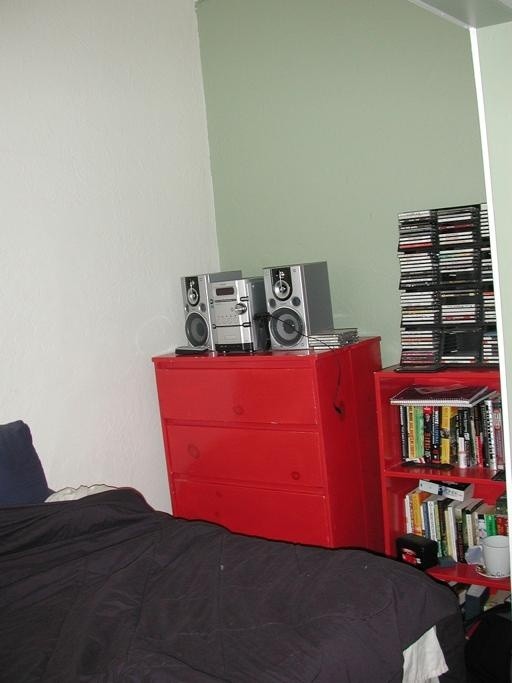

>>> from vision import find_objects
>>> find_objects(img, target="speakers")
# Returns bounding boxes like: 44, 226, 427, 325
181, 270, 242, 352
263, 261, 334, 350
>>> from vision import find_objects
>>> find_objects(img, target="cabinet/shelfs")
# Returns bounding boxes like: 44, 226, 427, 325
372, 365, 510, 601
151, 336, 386, 554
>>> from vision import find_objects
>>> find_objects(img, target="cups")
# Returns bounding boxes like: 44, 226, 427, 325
481, 534, 510, 577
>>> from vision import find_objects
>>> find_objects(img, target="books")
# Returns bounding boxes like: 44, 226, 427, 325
390, 385, 512, 623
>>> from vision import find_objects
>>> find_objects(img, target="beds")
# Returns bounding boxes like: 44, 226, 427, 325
0, 419, 468, 682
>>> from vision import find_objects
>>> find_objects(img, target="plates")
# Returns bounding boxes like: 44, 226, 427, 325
475, 563, 510, 580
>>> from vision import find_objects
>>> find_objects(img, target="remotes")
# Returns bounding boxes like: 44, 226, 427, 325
175, 346, 208, 354
